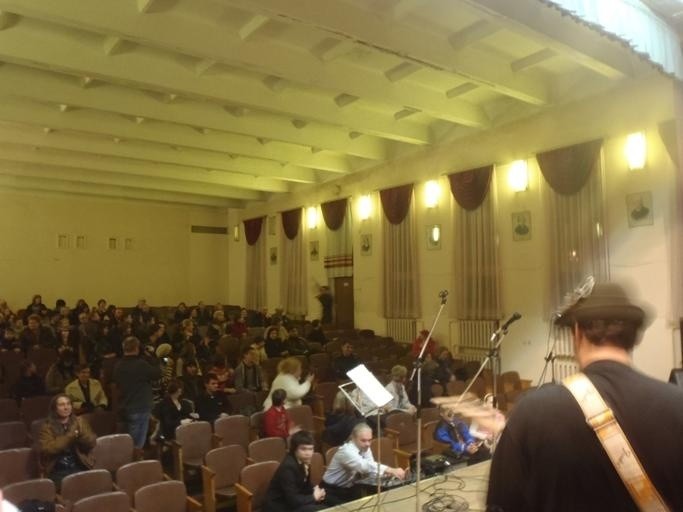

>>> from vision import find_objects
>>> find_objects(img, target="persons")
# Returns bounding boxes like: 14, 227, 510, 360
252, 308, 271, 328
12, 359, 45, 400
330, 340, 375, 378
112, 337, 166, 449
232, 348, 270, 395
263, 316, 290, 341
486, 285, 683, 512
45, 347, 81, 391
65, 364, 108, 411
383, 366, 418, 417
34, 393, 98, 494
269, 306, 289, 324
156, 302, 255, 380
155, 382, 200, 441
320, 390, 382, 447
0, 299, 26, 351
195, 374, 235, 424
19, 315, 58, 354
263, 357, 315, 412
318, 286, 334, 324
51, 299, 167, 361
408, 330, 437, 361
29, 308, 49, 324
307, 319, 327, 343
262, 389, 301, 439
410, 352, 440, 407
433, 404, 490, 466
260, 431, 329, 512
283, 327, 310, 354
319, 422, 406, 506
25, 295, 46, 317
264, 330, 288, 357
433, 347, 456, 382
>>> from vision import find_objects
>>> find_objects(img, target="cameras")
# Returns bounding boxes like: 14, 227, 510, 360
162, 357, 168, 362
139, 344, 154, 355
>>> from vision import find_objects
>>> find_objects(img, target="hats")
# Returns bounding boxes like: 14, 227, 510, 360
391, 365, 408, 381
554, 283, 645, 328
156, 343, 172, 358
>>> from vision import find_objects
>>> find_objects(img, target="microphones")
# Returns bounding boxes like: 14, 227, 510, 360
439, 289, 449, 304
501, 312, 523, 330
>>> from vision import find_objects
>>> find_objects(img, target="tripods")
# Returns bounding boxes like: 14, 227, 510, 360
359, 413, 389, 512
450, 348, 501, 410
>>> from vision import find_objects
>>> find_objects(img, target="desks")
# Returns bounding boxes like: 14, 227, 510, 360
316, 460, 494, 511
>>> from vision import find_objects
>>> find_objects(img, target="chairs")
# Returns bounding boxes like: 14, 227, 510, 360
93, 434, 134, 472
131, 481, 202, 512
76, 412, 116, 438
0, 479, 55, 512
0, 449, 33, 488
213, 416, 256, 449
309, 354, 335, 383
236, 462, 281, 511
293, 356, 309, 367
71, 492, 131, 512
245, 438, 286, 465
425, 420, 451, 454
270, 357, 284, 373
0, 421, 33, 451
55, 470, 118, 507
310, 343, 324, 353
173, 423, 223, 487
326, 342, 340, 355
32, 419, 41, 447
430, 361, 531, 413
341, 328, 430, 381
384, 413, 422, 470
326, 447, 342, 466
312, 383, 340, 417
250, 412, 265, 440
202, 444, 247, 511
0, 399, 21, 421
368, 437, 410, 488
308, 453, 326, 486
286, 406, 325, 439
21, 397, 52, 420
113, 460, 174, 496
